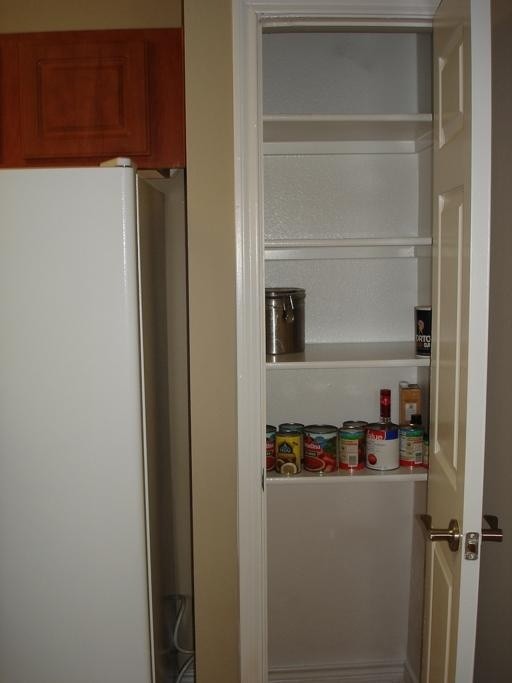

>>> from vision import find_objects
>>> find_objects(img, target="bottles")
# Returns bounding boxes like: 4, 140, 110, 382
378, 389, 393, 427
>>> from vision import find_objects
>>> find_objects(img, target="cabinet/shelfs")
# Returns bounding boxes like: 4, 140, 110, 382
236, 0, 504, 678
0, 27, 187, 169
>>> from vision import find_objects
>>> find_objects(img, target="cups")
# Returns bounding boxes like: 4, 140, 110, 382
415, 305, 432, 359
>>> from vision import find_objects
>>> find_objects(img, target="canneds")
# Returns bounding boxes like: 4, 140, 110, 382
399, 424, 425, 467
266, 420, 369, 475
424, 430, 429, 468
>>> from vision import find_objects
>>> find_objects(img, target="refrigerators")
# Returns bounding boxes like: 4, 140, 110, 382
0, 166, 193, 682
